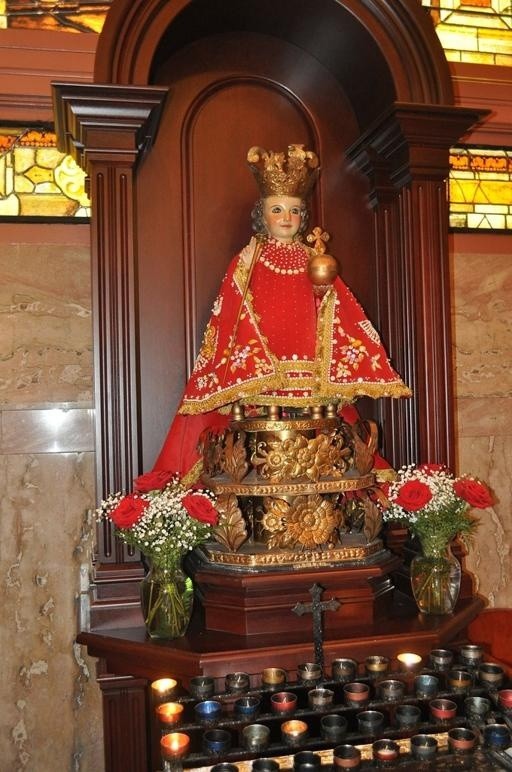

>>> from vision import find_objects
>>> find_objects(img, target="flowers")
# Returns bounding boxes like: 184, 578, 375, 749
95, 463, 229, 638
374, 464, 502, 612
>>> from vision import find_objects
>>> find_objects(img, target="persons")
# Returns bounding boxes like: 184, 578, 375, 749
174, 139, 415, 422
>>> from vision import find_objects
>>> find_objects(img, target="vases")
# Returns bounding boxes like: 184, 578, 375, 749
141, 547, 198, 636
408, 529, 462, 612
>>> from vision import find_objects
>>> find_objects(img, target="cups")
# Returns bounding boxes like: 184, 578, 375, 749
152, 644, 512, 771
